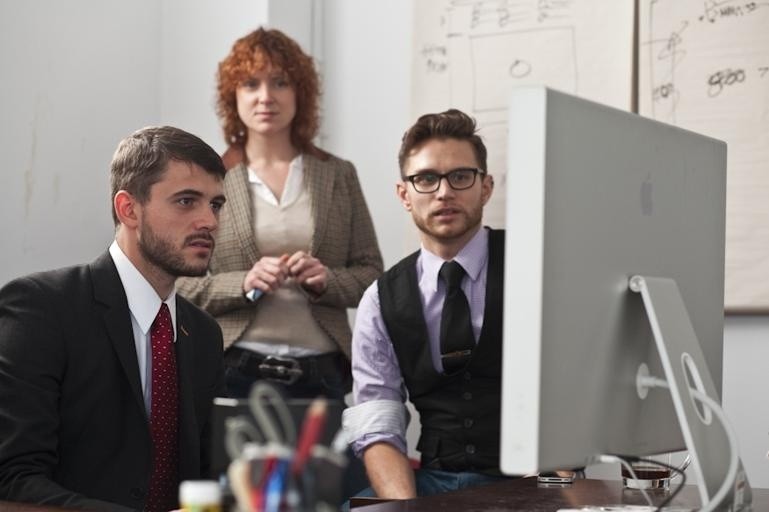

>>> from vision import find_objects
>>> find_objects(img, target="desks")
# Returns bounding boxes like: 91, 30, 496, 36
345, 476, 769, 512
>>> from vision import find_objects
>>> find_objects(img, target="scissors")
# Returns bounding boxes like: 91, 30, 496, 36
224, 377, 314, 512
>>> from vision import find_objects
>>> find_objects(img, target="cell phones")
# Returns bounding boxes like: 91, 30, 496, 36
537, 472, 576, 483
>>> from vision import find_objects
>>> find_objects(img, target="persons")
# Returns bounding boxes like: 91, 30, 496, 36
175, 24, 387, 459
0, 124, 229, 512
339, 106, 586, 510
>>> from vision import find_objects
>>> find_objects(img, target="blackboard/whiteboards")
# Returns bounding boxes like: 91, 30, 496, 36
410, 0, 769, 316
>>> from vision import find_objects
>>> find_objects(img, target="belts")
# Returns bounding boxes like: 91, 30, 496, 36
228, 344, 343, 386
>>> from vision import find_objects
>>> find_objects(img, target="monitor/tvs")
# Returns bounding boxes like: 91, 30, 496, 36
499, 85, 753, 511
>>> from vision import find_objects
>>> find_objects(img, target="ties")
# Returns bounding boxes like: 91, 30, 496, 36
146, 300, 181, 511
437, 262, 474, 380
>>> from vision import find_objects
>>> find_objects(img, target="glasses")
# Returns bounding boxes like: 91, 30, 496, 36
405, 166, 486, 194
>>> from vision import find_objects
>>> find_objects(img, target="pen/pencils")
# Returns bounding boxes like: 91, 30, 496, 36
264, 471, 284, 512
290, 395, 329, 478
